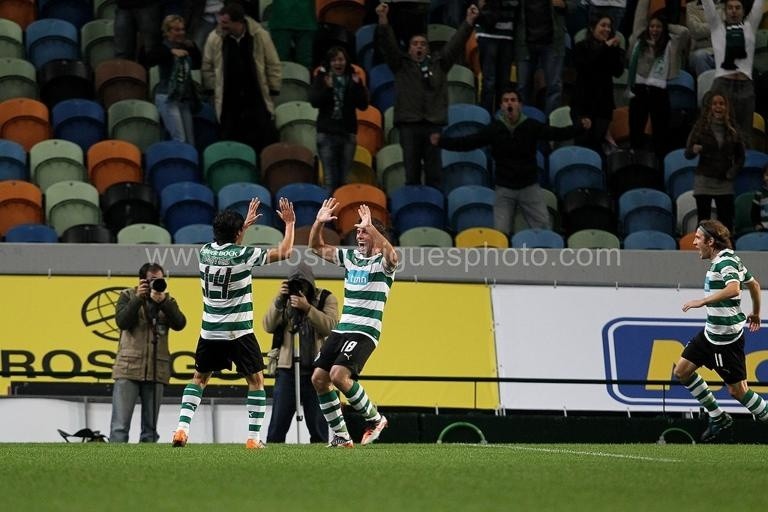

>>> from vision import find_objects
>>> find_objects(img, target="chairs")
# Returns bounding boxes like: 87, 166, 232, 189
0, 0, 768, 250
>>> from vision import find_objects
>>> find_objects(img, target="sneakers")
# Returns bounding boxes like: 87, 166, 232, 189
325, 435, 353, 448
361, 415, 387, 446
700, 411, 733, 442
171, 430, 188, 447
246, 439, 265, 448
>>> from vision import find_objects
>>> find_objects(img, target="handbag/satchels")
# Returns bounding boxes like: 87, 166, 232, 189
267, 348, 279, 375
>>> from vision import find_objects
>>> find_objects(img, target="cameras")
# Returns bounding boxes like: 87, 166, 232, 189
286, 280, 303, 298
145, 278, 167, 296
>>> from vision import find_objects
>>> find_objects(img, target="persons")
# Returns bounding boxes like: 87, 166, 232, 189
109, 263, 187, 442
308, 197, 398, 450
262, 261, 339, 443
672, 219, 768, 441
110, 1, 768, 248
171, 196, 296, 454
